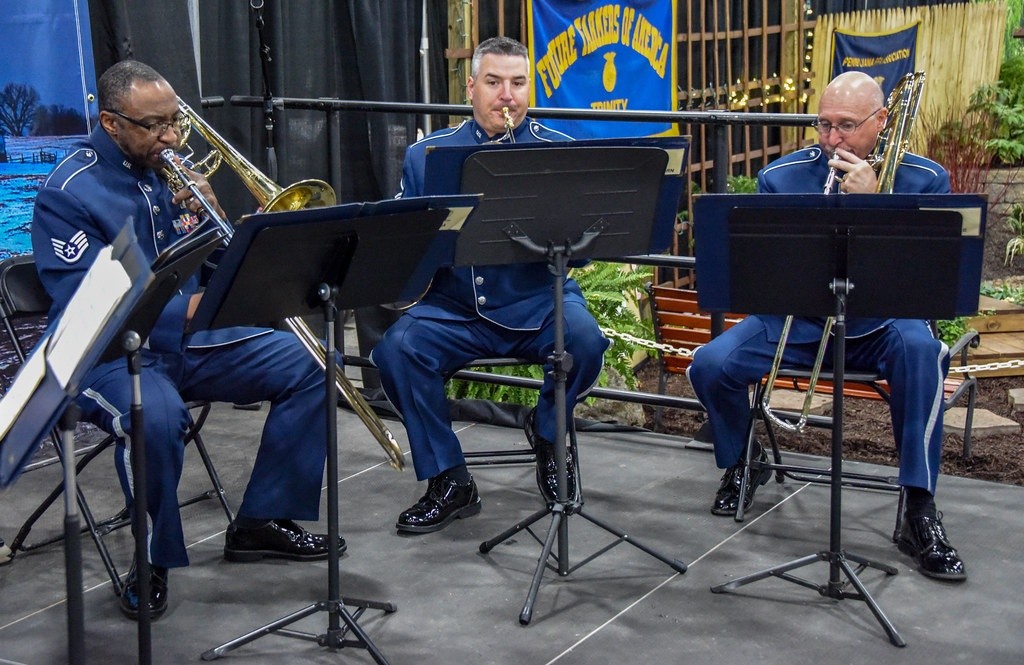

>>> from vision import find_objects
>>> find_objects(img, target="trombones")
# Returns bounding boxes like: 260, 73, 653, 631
760, 70, 927, 433
158, 95, 408, 471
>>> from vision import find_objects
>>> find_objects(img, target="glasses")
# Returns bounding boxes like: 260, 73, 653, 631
811, 107, 882, 134
104, 109, 189, 134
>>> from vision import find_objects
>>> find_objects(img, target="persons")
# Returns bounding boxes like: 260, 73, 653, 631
368, 38, 610, 532
685, 70, 965, 579
34, 61, 346, 621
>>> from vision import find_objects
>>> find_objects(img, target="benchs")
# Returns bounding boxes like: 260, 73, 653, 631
650, 285, 980, 463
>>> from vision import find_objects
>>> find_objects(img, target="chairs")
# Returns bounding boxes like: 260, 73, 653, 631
0, 259, 240, 599
376, 351, 584, 502
736, 367, 906, 535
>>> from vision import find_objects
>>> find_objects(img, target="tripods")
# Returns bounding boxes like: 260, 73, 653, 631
190, 193, 483, 665
692, 193, 990, 649
424, 133, 692, 625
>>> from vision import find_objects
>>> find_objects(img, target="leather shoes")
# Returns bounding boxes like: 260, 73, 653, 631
709, 439, 771, 514
524, 406, 576, 503
896, 511, 966, 579
121, 555, 169, 618
395, 473, 481, 533
223, 521, 347, 561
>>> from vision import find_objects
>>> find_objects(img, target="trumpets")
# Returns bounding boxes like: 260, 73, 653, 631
480, 107, 516, 144
